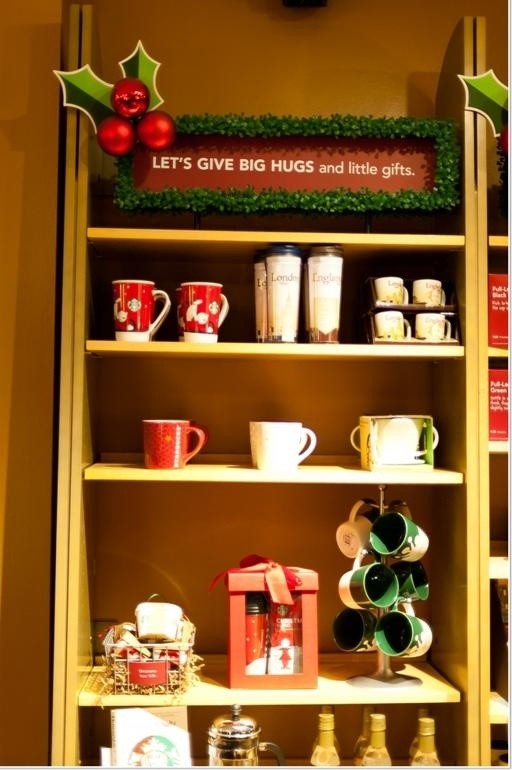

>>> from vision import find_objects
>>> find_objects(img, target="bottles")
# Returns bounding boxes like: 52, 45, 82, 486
309, 705, 441, 766
205, 704, 286, 766
142, 418, 209, 469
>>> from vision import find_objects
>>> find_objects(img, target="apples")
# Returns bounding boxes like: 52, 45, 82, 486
110, 76, 150, 119
95, 116, 135, 157
135, 110, 177, 152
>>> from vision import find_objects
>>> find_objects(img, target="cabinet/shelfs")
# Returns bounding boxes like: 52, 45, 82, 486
51, 1, 475, 766
475, 13, 508, 764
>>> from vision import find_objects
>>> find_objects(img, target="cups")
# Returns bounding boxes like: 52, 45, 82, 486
254, 243, 346, 344
374, 277, 452, 343
350, 414, 439, 472
111, 279, 230, 345
250, 421, 318, 472
332, 497, 434, 659
